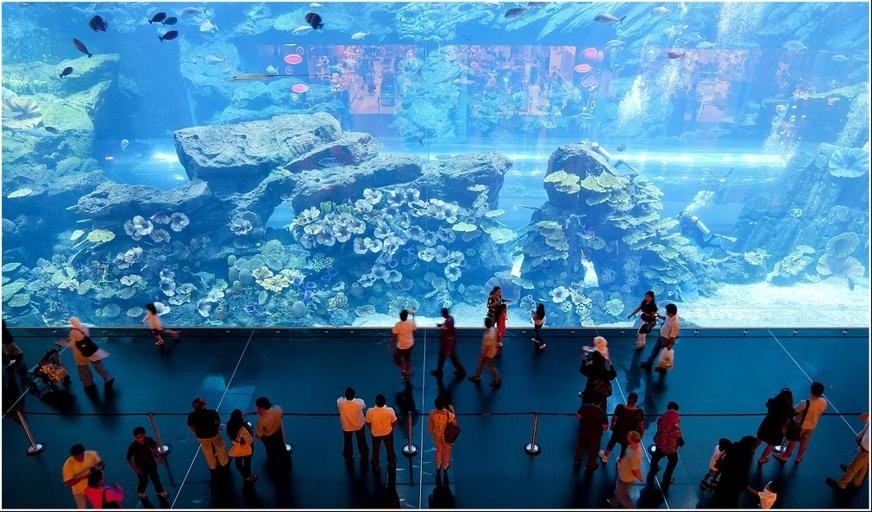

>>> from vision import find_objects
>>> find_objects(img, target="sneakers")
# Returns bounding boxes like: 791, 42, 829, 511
539, 344, 546, 349
156, 489, 169, 497
825, 476, 843, 494
156, 339, 166, 346
493, 379, 501, 389
531, 338, 540, 343
655, 366, 666, 372
839, 463, 848, 472
136, 485, 146, 498
639, 359, 653, 365
661, 476, 675, 484
699, 479, 718, 491
430, 369, 482, 384
599, 449, 608, 463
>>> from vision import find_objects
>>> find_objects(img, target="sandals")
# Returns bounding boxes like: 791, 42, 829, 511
760, 453, 804, 464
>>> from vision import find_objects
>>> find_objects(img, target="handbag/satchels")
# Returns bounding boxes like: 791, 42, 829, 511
75, 335, 100, 357
102, 500, 121, 508
714, 458, 723, 468
784, 421, 801, 441
444, 423, 461, 443
228, 440, 252, 457
638, 323, 651, 334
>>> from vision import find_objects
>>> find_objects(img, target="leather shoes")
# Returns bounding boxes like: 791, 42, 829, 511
245, 474, 257, 483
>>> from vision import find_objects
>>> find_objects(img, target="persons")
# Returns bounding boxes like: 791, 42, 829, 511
2, 320, 24, 369
62, 443, 125, 508
337, 387, 398, 472
140, 302, 182, 346
187, 396, 291, 480
825, 413, 870, 496
67, 316, 116, 388
428, 392, 456, 484
128, 427, 169, 497
699, 382, 828, 509
387, 284, 681, 383
573, 381, 682, 509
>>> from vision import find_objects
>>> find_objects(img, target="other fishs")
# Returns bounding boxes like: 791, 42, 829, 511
59, 67, 74, 78
148, 2, 370, 81
848, 278, 854, 291
121, 139, 129, 152
89, 15, 108, 32
72, 38, 93, 58
504, 1, 871, 62
618, 143, 626, 151
